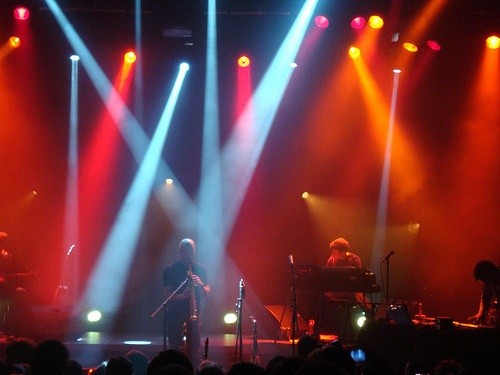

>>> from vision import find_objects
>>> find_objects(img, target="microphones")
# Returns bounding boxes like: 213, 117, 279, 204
381, 250, 394, 263
203, 338, 208, 356
239, 281, 246, 298
185, 260, 195, 273
67, 244, 75, 255
288, 254, 295, 273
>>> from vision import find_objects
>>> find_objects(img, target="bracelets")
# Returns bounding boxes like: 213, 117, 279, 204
198, 283, 205, 288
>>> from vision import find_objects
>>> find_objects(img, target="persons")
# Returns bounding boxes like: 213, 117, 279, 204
465, 260, 500, 328
163, 238, 210, 374
312, 237, 373, 342
0, 320, 500, 375
0, 231, 20, 299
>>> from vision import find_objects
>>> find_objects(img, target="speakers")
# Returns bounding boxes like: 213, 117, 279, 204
257, 304, 309, 340
